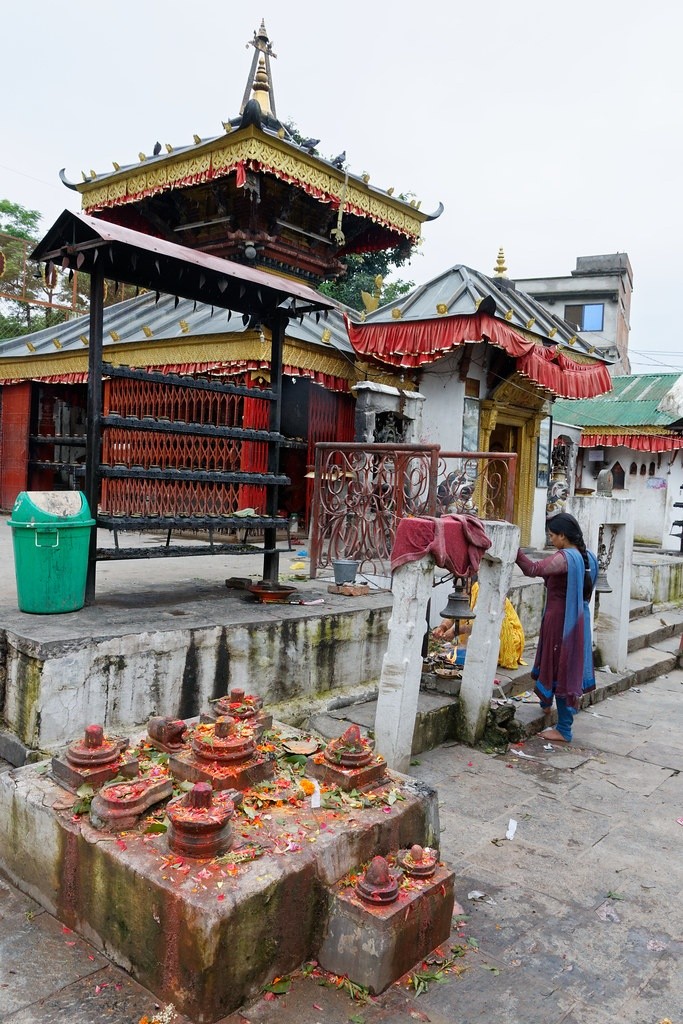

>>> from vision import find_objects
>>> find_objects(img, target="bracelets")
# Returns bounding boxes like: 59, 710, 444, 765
439, 624, 448, 632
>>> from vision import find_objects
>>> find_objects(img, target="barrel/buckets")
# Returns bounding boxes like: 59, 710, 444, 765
332, 559, 361, 583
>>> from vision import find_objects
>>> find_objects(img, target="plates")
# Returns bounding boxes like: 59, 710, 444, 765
248, 585, 299, 601
435, 669, 459, 679
575, 487, 595, 494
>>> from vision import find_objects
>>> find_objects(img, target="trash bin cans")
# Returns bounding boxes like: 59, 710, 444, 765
6, 487, 95, 615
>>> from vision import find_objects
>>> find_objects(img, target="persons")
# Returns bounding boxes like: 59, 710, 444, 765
515, 512, 599, 743
431, 570, 527, 670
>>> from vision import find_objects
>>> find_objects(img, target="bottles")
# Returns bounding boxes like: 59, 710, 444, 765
98, 511, 287, 528
29, 433, 87, 442
35, 460, 85, 472
99, 463, 288, 484
108, 411, 281, 441
102, 360, 274, 399
289, 512, 299, 533
